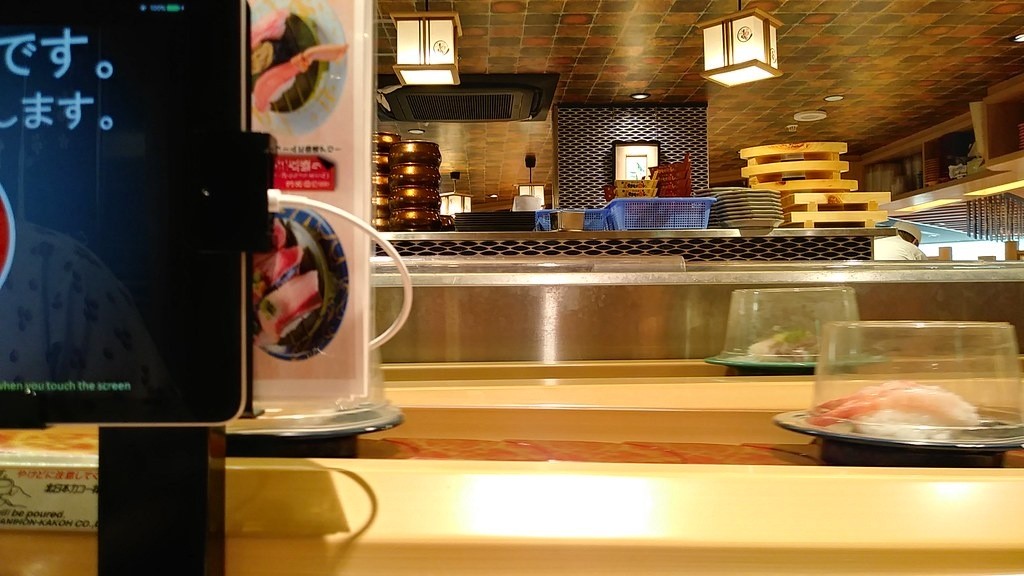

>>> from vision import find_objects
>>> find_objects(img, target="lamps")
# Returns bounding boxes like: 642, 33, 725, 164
518, 154, 545, 210
389, 0, 464, 85
439, 172, 473, 216
695, 0, 785, 89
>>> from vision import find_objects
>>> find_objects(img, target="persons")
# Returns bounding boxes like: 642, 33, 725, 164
874, 222, 928, 260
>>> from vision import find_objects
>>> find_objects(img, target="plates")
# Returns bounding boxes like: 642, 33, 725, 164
703, 357, 888, 376
454, 210, 537, 231
225, 404, 407, 441
1016, 123, 1024, 150
772, 411, 1024, 459
693, 186, 786, 228
923, 158, 941, 187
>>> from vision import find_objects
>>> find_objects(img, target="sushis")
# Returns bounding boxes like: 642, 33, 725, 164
256, 270, 321, 340
253, 44, 347, 112
252, 217, 287, 265
246, 9, 290, 50
253, 248, 305, 296
812, 387, 978, 441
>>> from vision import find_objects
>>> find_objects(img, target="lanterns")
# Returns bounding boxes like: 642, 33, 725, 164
512, 168, 546, 209
389, 0, 463, 85
439, 180, 475, 217
696, 0, 785, 88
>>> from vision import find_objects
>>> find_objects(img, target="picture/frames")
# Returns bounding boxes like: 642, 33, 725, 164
612, 140, 660, 187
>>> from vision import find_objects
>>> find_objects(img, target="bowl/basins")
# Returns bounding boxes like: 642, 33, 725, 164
796, 321, 1024, 442
714, 287, 865, 363
863, 156, 922, 198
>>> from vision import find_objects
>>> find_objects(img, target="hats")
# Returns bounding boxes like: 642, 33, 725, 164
893, 221, 922, 244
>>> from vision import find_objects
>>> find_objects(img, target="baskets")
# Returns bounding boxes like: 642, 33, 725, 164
603, 195, 716, 231
533, 209, 610, 231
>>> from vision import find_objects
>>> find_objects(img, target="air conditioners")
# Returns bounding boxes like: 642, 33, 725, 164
376, 73, 561, 123
